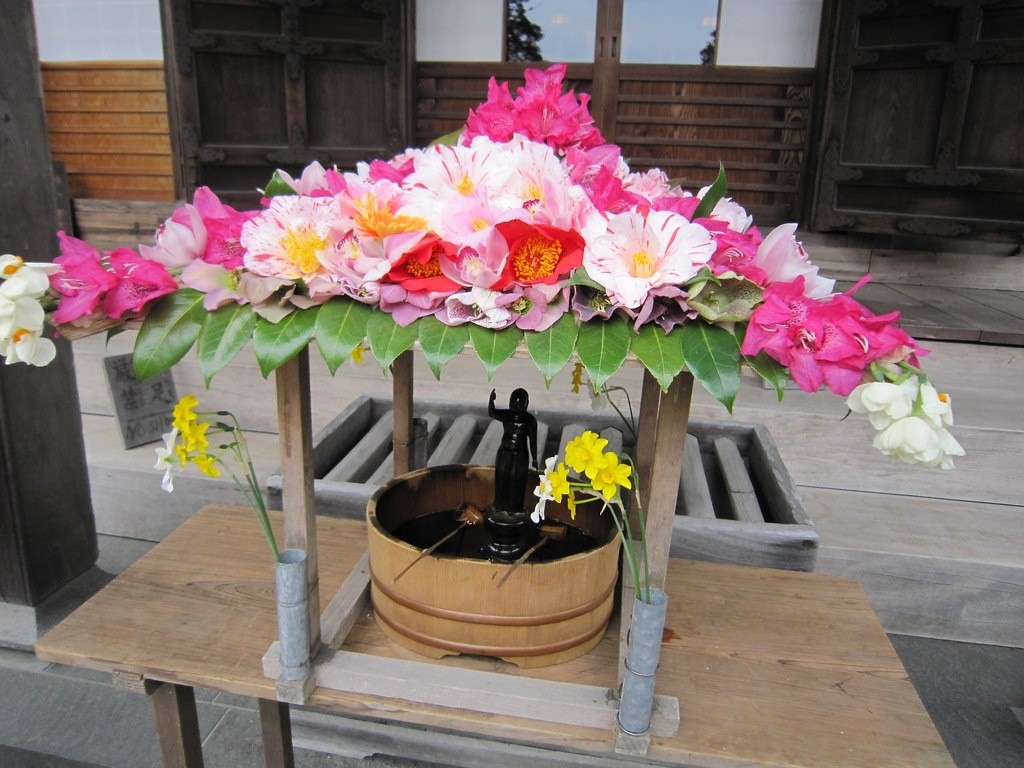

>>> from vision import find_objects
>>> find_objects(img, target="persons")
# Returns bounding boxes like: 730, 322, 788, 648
488, 387, 538, 515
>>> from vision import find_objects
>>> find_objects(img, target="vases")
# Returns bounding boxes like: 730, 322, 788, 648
618, 588, 669, 736
273, 548, 310, 680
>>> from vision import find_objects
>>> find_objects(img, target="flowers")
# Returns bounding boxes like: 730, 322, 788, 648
529, 432, 649, 603
153, 394, 282, 565
0, 62, 966, 472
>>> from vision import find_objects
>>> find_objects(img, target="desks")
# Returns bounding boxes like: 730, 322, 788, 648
35, 503, 960, 768
